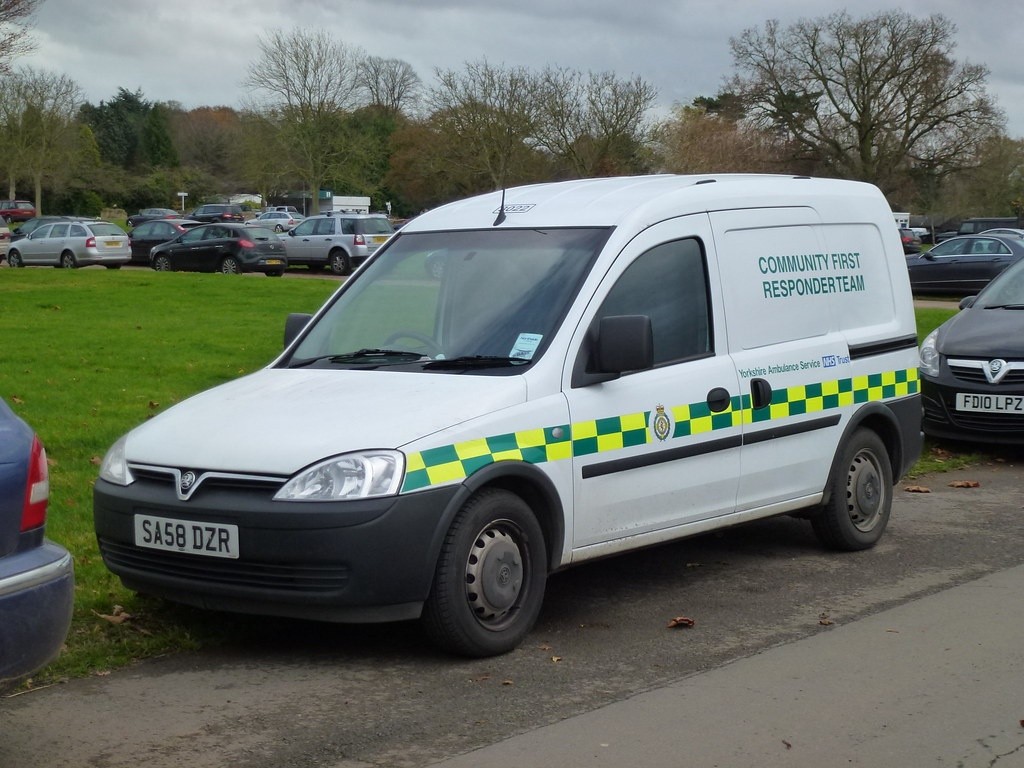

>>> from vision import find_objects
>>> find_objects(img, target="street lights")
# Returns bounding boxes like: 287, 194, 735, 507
178, 193, 188, 219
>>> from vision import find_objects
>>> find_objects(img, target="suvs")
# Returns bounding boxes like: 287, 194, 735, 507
5, 220, 131, 270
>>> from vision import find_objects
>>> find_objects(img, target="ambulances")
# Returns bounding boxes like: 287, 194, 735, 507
91, 173, 925, 656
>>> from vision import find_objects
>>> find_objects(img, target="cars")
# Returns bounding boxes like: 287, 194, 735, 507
0, 396, 74, 677
919, 254, 1024, 445
0, 215, 11, 264
10, 216, 95, 242
149, 223, 287, 277
127, 208, 183, 227
127, 218, 213, 264
275, 214, 397, 276
897, 229, 922, 254
0, 200, 35, 224
952, 228, 1024, 256
255, 206, 297, 218
905, 234, 1024, 294
184, 204, 245, 223
244, 211, 305, 233
934, 217, 1018, 244
